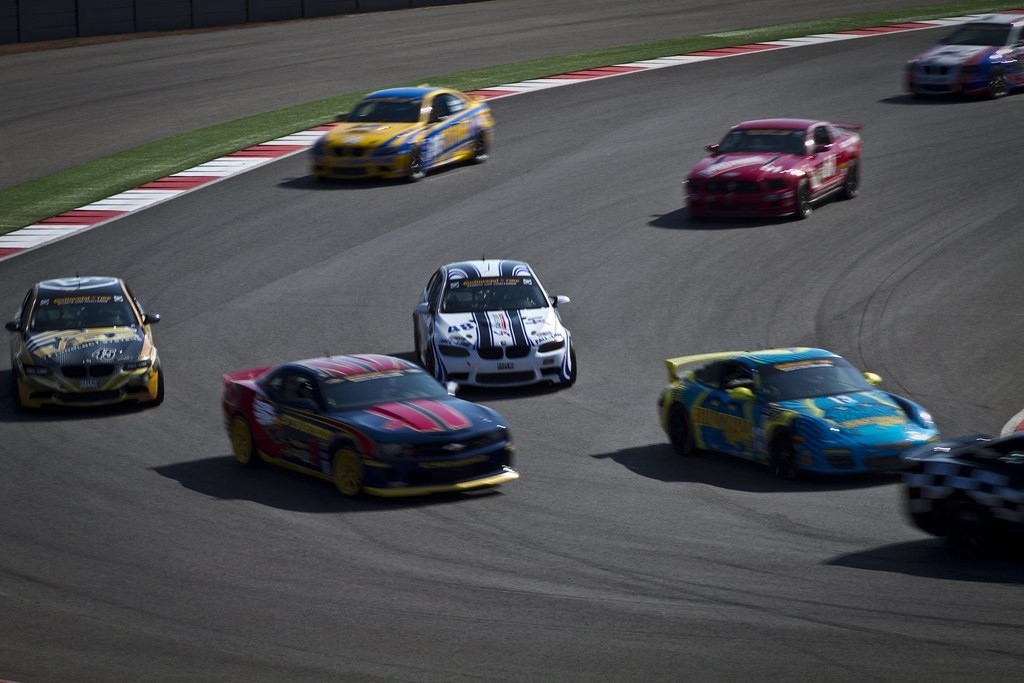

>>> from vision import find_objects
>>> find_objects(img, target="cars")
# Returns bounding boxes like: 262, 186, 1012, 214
902, 7, 1024, 100
409, 257, 578, 396
898, 430, 1024, 562
683, 117, 863, 219
307, 83, 497, 183
219, 350, 523, 503
4, 275, 166, 414
656, 344, 943, 487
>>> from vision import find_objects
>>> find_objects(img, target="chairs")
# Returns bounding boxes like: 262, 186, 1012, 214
445, 292, 473, 314
